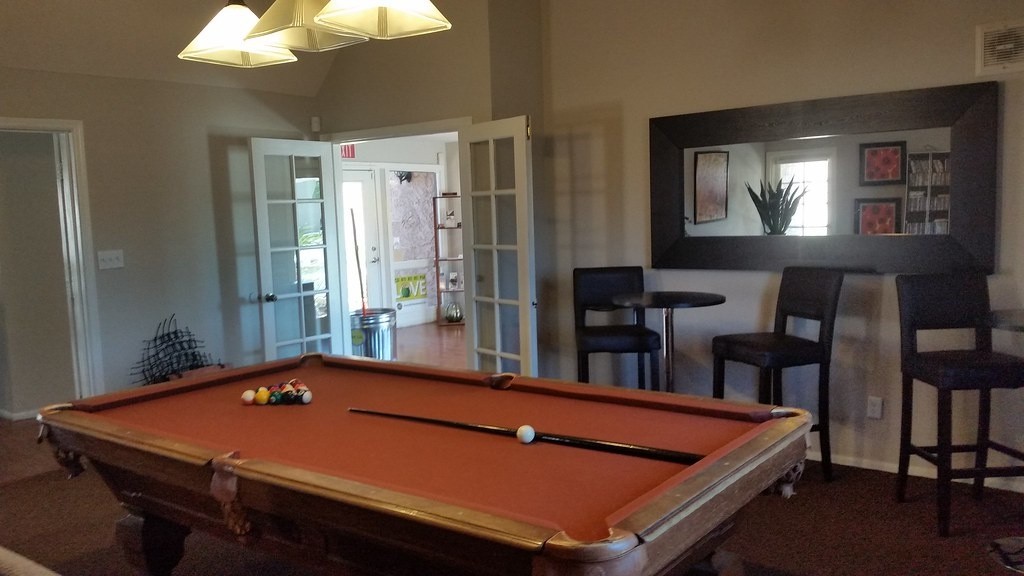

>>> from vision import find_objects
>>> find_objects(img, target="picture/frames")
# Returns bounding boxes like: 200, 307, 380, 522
859, 141, 907, 185
695, 151, 730, 225
854, 197, 900, 234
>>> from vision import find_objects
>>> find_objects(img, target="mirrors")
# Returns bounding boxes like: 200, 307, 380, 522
650, 81, 999, 274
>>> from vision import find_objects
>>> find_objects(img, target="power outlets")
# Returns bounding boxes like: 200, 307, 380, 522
868, 397, 883, 419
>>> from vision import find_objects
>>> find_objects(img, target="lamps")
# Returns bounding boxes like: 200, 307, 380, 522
178, 0, 452, 68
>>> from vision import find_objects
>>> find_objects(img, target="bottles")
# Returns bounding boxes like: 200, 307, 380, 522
439, 272, 446, 290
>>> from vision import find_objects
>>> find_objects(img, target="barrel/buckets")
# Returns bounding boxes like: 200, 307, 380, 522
349, 308, 397, 363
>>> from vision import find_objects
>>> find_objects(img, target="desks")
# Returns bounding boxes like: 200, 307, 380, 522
36, 353, 814, 576
614, 291, 725, 393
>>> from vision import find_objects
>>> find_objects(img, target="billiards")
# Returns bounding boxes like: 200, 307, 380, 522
282, 390, 297, 403
242, 390, 256, 404
255, 378, 307, 405
268, 392, 283, 404
516, 424, 535, 444
296, 389, 313, 404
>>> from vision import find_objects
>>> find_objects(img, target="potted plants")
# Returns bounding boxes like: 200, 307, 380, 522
745, 174, 808, 236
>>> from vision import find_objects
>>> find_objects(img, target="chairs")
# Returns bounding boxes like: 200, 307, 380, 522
712, 267, 844, 480
574, 266, 662, 391
894, 268, 1024, 539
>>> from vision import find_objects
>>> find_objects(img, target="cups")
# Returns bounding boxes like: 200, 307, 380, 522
402, 287, 409, 297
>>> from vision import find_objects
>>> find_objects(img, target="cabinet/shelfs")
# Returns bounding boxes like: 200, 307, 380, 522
903, 151, 951, 235
433, 196, 466, 327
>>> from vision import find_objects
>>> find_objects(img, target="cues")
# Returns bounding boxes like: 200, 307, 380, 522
347, 407, 705, 465
